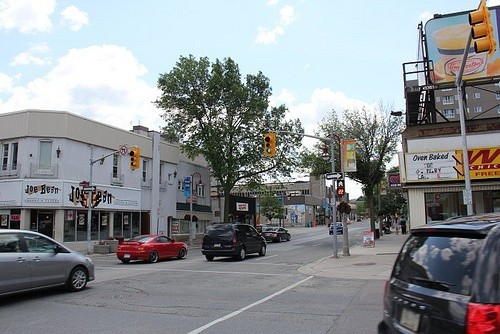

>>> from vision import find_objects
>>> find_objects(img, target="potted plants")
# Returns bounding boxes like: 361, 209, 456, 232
94, 237, 119, 254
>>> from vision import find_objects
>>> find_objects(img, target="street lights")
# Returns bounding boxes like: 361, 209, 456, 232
189, 172, 204, 246
322, 134, 350, 257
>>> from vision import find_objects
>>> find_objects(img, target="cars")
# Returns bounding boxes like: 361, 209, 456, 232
115, 234, 189, 264
261, 227, 291, 243
0, 228, 96, 297
328, 222, 343, 235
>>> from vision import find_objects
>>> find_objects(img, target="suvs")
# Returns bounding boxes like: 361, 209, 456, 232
377, 211, 500, 334
201, 222, 267, 261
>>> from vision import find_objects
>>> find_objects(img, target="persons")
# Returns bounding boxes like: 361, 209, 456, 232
374, 217, 381, 239
383, 223, 390, 234
399, 215, 406, 234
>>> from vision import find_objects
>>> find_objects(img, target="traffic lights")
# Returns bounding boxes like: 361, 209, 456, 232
264, 133, 276, 158
336, 179, 345, 195
468, 0, 497, 61
92, 194, 100, 207
79, 194, 89, 208
129, 148, 140, 170
452, 154, 464, 176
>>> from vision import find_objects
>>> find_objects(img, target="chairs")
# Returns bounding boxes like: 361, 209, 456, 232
6, 241, 18, 252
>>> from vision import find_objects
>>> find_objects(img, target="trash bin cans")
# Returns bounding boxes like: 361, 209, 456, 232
376, 229, 380, 238
255, 226, 262, 233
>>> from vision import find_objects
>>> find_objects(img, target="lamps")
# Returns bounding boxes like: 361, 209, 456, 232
173, 169, 177, 178
390, 110, 422, 117
56, 146, 61, 158
100, 154, 105, 164
